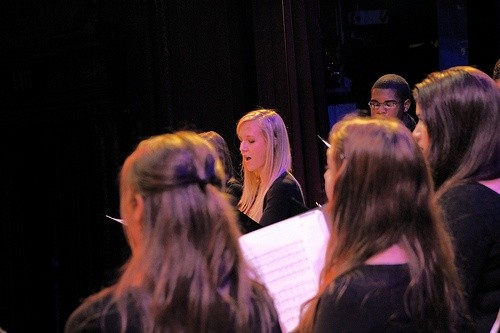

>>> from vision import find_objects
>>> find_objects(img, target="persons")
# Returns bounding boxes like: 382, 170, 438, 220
199, 132, 242, 206
66, 133, 282, 332
412, 61, 500, 333
369, 75, 416, 131
231, 108, 307, 227
296, 119, 474, 332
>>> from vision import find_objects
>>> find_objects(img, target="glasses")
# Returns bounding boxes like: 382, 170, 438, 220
368, 99, 406, 109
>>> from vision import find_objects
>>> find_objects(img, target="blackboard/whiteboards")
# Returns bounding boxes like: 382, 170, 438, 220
240, 204, 331, 333
